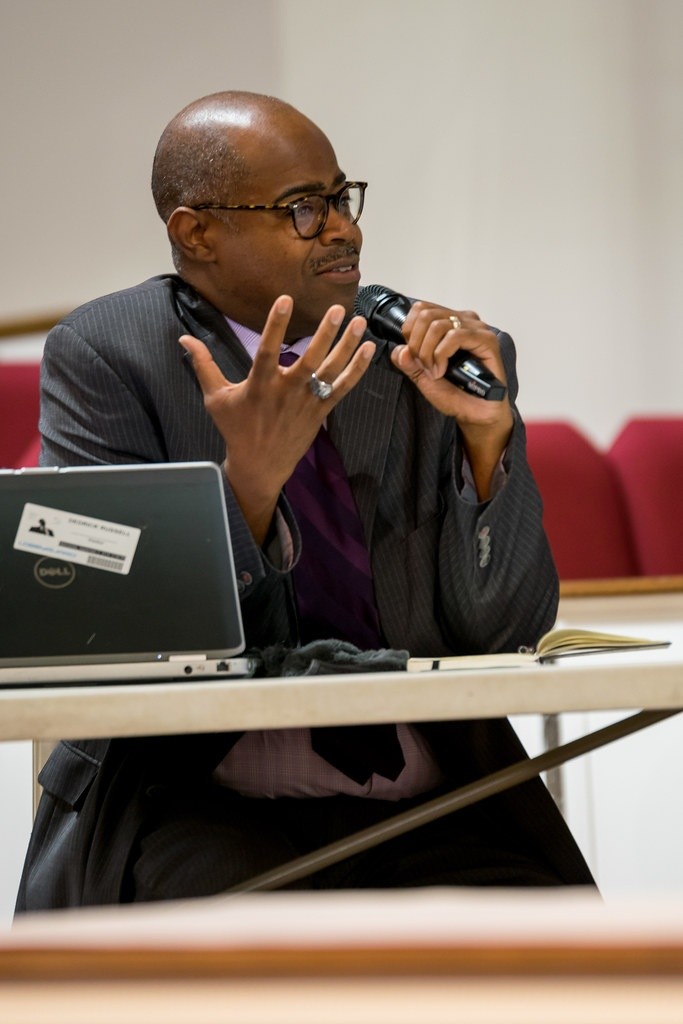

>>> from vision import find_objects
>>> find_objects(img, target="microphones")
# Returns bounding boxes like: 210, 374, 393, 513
354, 283, 506, 401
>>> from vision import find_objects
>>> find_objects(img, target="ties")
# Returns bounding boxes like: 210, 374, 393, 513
279, 349, 404, 785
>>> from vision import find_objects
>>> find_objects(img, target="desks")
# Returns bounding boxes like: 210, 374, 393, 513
0, 627, 683, 915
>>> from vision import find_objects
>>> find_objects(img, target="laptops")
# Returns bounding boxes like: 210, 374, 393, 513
0, 460, 261, 684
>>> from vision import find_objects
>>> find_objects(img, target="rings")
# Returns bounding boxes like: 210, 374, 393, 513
310, 372, 334, 400
449, 315, 462, 329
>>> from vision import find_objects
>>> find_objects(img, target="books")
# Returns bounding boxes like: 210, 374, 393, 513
406, 627, 671, 673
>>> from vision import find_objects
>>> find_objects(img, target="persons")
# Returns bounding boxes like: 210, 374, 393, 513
13, 90, 599, 915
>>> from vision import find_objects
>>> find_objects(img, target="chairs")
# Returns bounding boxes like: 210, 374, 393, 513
0, 360, 683, 606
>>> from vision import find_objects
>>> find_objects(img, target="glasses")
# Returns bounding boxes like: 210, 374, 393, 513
187, 180, 368, 239
